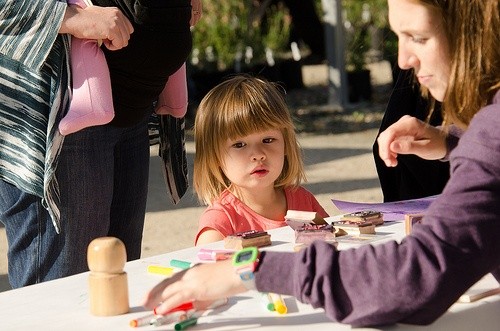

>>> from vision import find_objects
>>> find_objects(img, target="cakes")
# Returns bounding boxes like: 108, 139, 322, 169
332, 210, 385, 235
283, 209, 348, 242
223, 229, 272, 249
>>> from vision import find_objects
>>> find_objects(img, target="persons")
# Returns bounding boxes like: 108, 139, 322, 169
0, 0, 202, 291
144, 0, 500, 328
193, 76, 331, 248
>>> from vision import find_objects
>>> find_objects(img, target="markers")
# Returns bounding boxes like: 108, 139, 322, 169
261, 293, 287, 315
138, 256, 194, 276
130, 296, 229, 331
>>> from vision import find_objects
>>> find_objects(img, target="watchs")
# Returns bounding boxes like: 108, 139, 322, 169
231, 247, 265, 292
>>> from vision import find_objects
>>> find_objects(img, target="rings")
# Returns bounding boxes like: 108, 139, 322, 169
192, 10, 201, 17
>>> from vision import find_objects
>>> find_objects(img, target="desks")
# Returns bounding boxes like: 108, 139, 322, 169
0, 212, 500, 331
